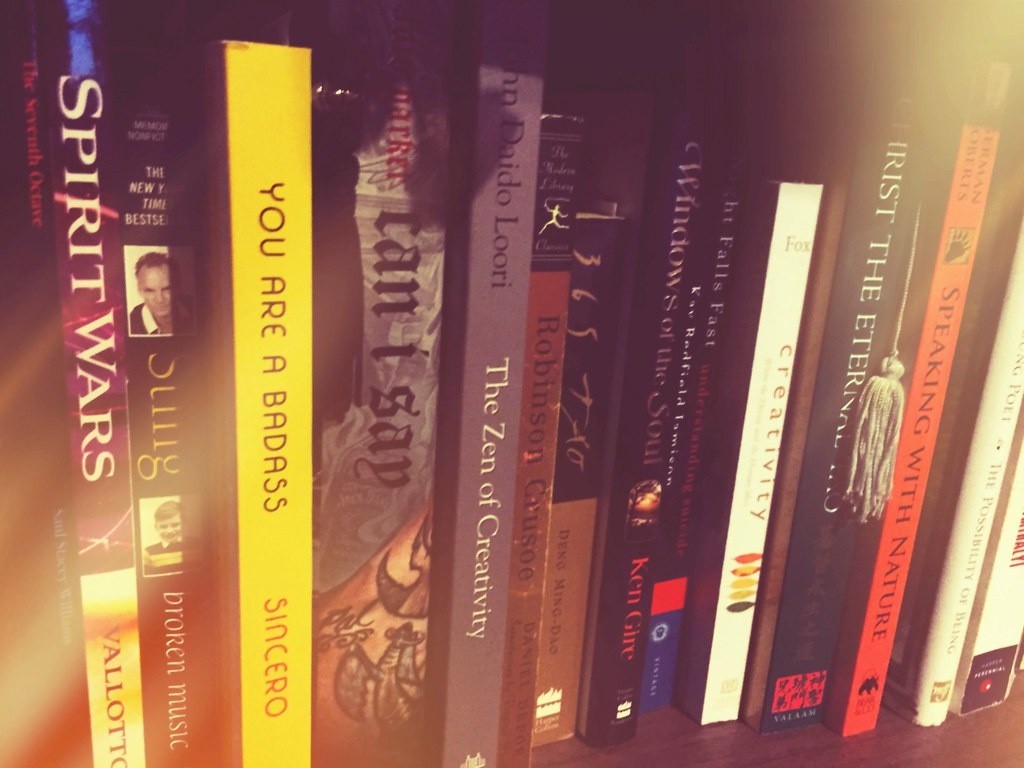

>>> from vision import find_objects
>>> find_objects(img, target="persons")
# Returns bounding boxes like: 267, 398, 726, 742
131, 251, 194, 336
144, 501, 201, 574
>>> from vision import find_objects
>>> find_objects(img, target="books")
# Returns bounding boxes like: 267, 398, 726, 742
0, 0, 1024, 768
